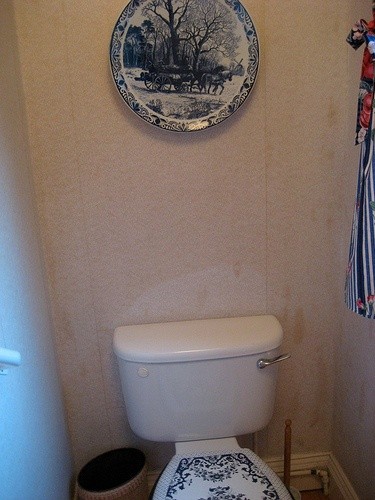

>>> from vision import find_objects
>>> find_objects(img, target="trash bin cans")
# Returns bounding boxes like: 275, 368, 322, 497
76, 447, 151, 500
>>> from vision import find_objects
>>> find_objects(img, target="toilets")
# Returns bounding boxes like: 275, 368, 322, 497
113, 314, 294, 499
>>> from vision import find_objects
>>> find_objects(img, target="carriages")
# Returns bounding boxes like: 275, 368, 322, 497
134, 65, 232, 94
